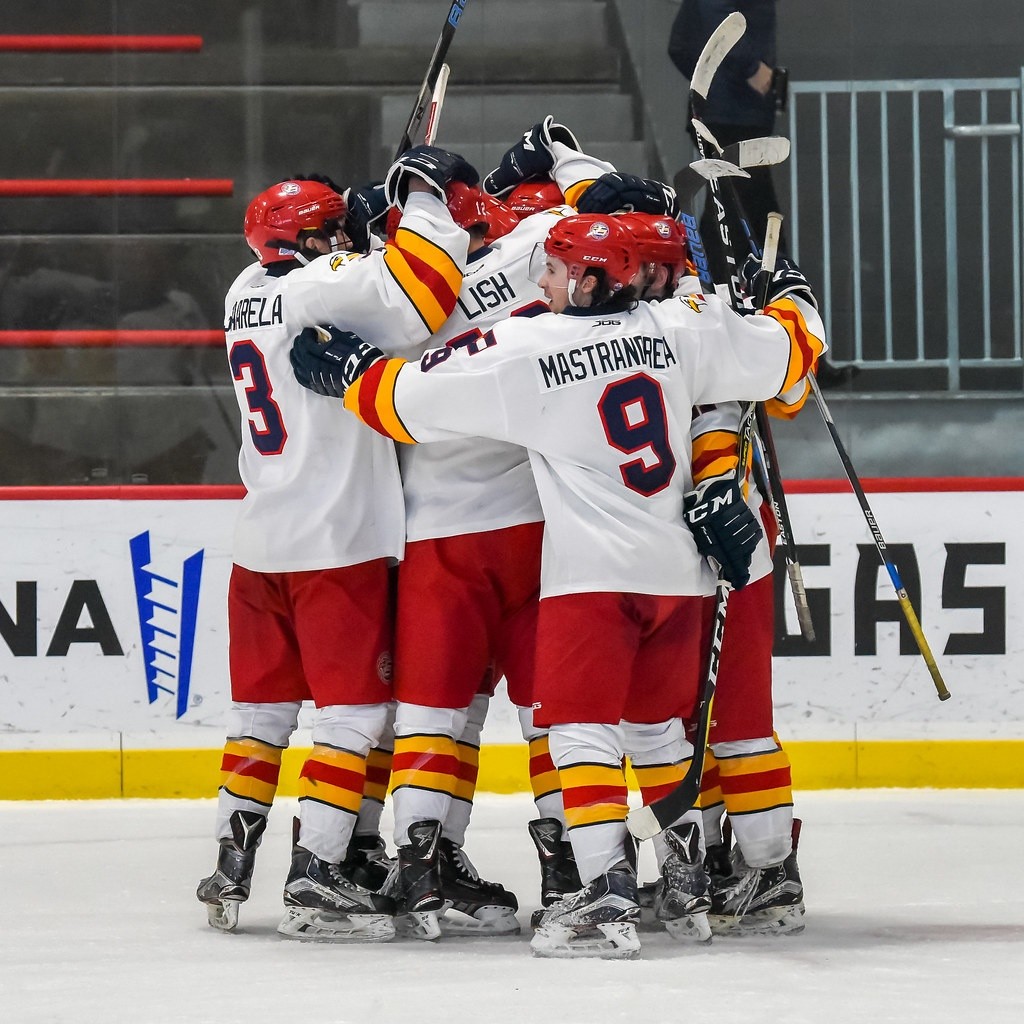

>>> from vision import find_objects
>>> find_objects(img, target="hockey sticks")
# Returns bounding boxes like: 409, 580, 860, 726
684, 11, 952, 703
390, 0, 467, 162
627, 212, 784, 843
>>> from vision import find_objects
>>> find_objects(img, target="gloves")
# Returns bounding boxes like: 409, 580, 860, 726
576, 172, 682, 223
742, 252, 819, 312
289, 322, 388, 398
283, 173, 389, 255
384, 144, 481, 214
482, 115, 584, 197
681, 469, 764, 591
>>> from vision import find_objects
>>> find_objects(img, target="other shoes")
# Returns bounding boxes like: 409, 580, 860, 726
816, 355, 861, 389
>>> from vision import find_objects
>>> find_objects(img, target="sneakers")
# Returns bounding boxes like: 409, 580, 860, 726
345, 833, 404, 901
197, 811, 267, 931
440, 836, 521, 937
707, 818, 806, 936
277, 816, 396, 943
373, 818, 445, 941
528, 817, 587, 906
655, 821, 712, 942
638, 817, 732, 908
528, 831, 642, 958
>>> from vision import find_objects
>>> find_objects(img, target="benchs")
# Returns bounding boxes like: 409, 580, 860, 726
0, 82, 383, 233
0, 0, 359, 86
0, 234, 257, 386
0, 386, 241, 486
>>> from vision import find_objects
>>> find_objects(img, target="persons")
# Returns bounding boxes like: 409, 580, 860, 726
668, 0, 865, 392
196, 146, 479, 941
388, 181, 585, 941
291, 212, 825, 957
284, 118, 817, 936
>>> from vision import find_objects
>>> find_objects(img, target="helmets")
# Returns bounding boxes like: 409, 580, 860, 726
616, 212, 685, 287
506, 182, 565, 220
243, 180, 349, 268
543, 213, 640, 292
384, 180, 520, 247
